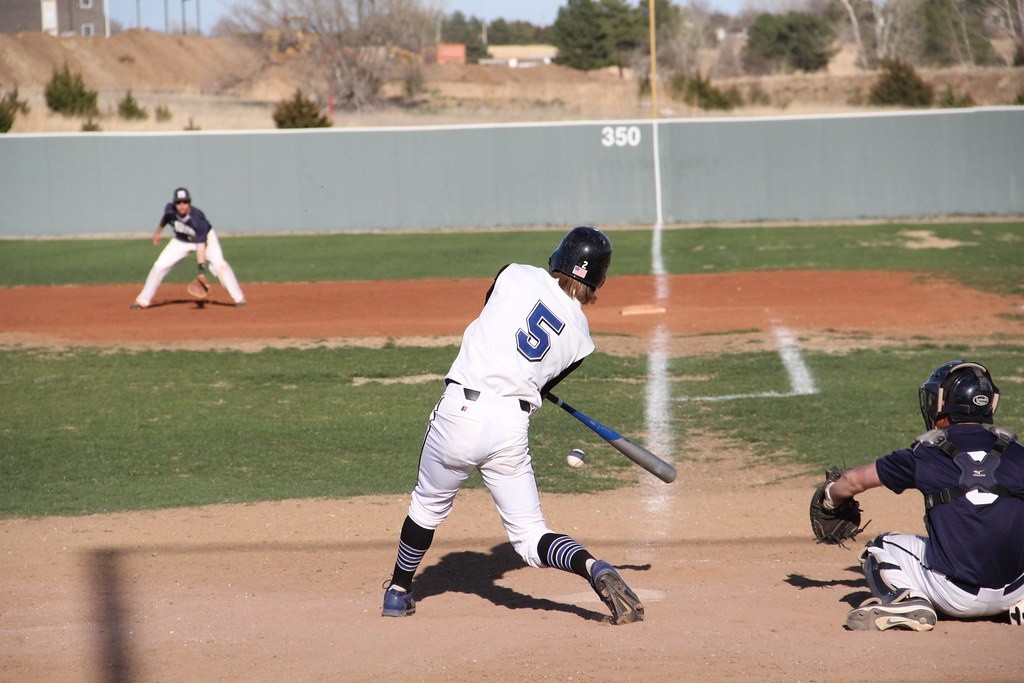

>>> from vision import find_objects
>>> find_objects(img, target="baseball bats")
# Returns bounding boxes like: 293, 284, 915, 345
543, 390, 678, 485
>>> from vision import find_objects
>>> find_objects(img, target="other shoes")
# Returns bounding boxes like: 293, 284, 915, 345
235, 302, 248, 307
131, 304, 144, 309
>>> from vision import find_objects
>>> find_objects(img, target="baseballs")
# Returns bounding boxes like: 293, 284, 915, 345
566, 448, 587, 469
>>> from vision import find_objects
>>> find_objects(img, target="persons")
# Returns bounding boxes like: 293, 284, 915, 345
129, 188, 245, 309
382, 226, 643, 625
809, 360, 1024, 632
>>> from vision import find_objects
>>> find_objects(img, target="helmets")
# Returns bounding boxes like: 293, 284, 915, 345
918, 360, 1000, 431
548, 226, 613, 289
174, 188, 192, 203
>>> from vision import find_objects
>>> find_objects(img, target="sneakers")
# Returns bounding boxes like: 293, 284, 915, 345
590, 559, 645, 625
381, 580, 416, 617
1009, 598, 1024, 625
844, 599, 938, 632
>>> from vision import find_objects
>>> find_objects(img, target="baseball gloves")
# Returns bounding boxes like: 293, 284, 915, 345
810, 466, 863, 545
186, 273, 212, 301
